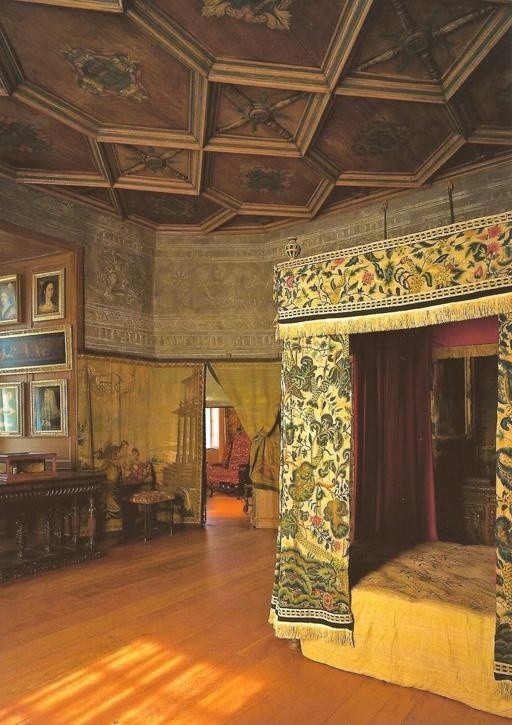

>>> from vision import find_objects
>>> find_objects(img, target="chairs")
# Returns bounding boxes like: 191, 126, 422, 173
8, 453, 69, 559
208, 427, 251, 513
118, 460, 176, 543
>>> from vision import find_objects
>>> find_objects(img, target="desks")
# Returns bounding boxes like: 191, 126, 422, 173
0, 471, 107, 583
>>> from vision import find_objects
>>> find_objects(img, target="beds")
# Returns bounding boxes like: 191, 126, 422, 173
267, 212, 512, 724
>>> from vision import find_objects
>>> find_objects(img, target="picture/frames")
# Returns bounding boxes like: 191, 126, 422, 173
30, 267, 66, 321
0, 381, 26, 438
0, 273, 22, 326
29, 379, 69, 438
0, 324, 73, 376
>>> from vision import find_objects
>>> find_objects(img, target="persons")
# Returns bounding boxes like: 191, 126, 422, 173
97, 438, 130, 512
0, 283, 17, 321
0, 391, 17, 432
35, 281, 58, 314
121, 446, 144, 477
39, 388, 61, 430
